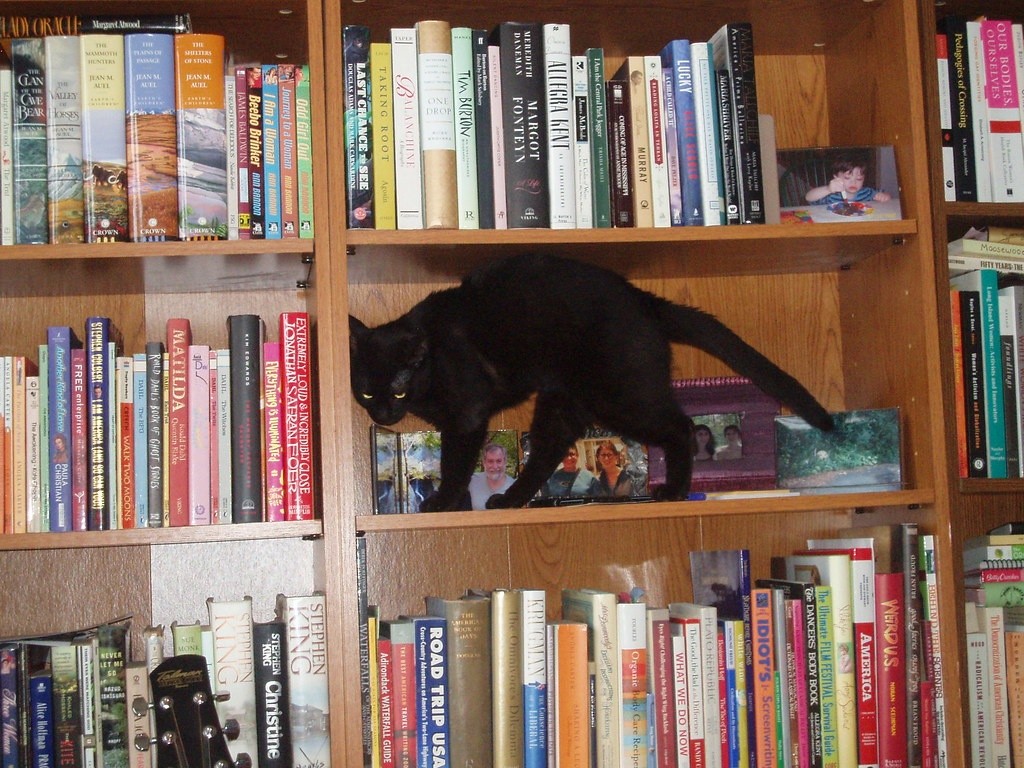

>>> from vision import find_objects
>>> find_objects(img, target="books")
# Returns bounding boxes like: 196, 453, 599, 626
948, 229, 1024, 478
369, 371, 909, 515
344, 16, 905, 226
0, 525, 1024, 768
0, 314, 319, 537
936, 17, 1024, 204
0, 12, 314, 244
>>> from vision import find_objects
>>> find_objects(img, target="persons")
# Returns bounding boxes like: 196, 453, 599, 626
807, 151, 889, 203
591, 443, 638, 501
549, 444, 602, 500
689, 424, 716, 461
714, 427, 743, 459
468, 445, 516, 511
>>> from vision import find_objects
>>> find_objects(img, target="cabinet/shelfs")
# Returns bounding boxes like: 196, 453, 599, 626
0, 0, 1024, 768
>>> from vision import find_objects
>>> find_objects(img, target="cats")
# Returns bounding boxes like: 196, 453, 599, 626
350, 256, 836, 515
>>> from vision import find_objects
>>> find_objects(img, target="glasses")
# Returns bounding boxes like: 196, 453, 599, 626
599, 453, 614, 457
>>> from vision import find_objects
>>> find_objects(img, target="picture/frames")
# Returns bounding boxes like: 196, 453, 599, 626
520, 426, 653, 509
645, 378, 781, 495
370, 424, 401, 515
